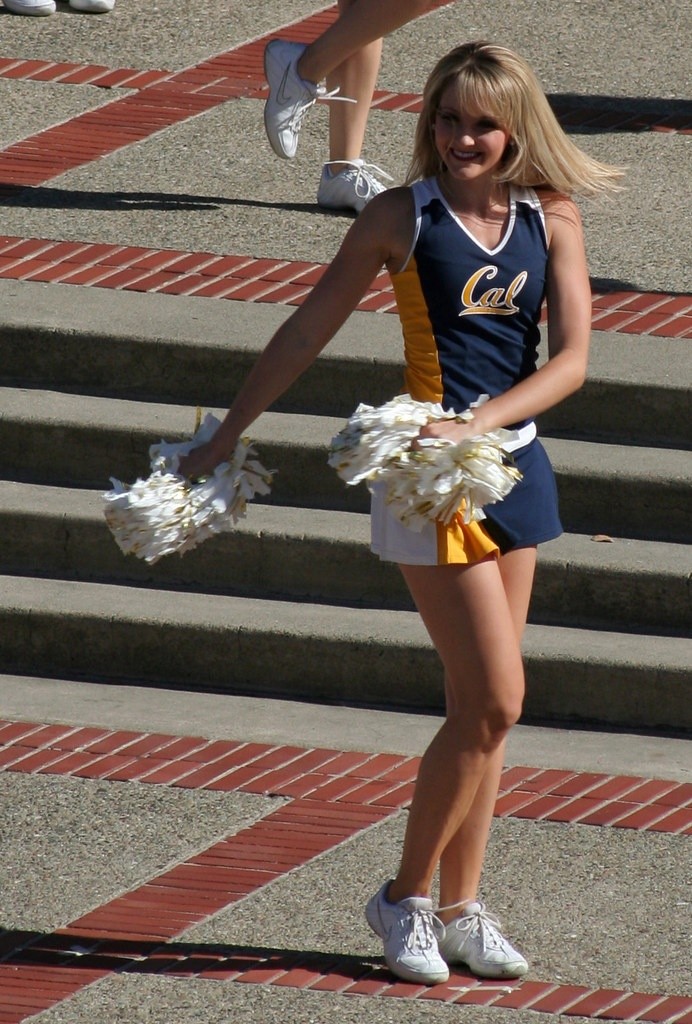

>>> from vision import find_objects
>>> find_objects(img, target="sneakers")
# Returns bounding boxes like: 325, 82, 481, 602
263, 38, 358, 159
317, 159, 396, 214
435, 901, 530, 979
363, 878, 450, 985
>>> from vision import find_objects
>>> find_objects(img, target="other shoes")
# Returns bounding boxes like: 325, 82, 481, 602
4, 0, 56, 16
70, 0, 114, 13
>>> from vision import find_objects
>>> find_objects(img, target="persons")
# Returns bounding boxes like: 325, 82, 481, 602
178, 40, 628, 985
263, 0, 459, 216
